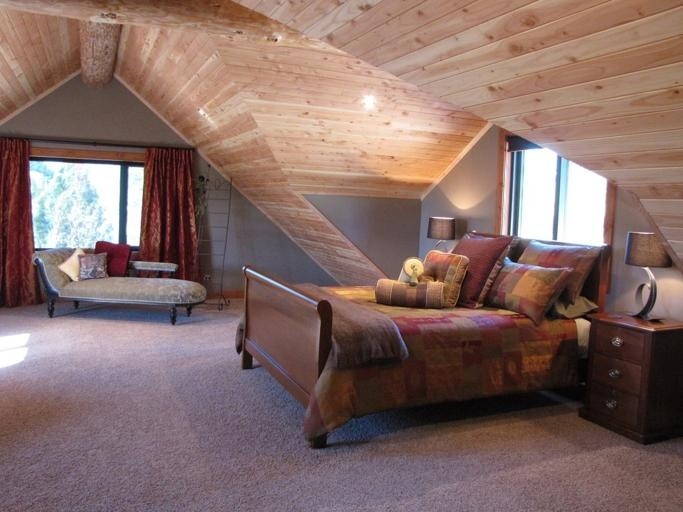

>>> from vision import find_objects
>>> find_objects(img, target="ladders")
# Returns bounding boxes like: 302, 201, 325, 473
198, 164, 232, 311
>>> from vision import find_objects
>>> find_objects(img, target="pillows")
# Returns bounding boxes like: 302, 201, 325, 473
77, 252, 110, 280
515, 237, 605, 305
54, 247, 87, 282
487, 255, 575, 329
373, 274, 445, 310
92, 240, 132, 277
443, 232, 519, 312
422, 248, 469, 311
396, 256, 425, 286
555, 294, 600, 321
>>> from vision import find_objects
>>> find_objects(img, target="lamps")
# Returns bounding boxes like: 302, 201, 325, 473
424, 214, 456, 246
621, 230, 674, 323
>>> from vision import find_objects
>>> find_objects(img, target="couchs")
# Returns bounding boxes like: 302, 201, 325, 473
31, 245, 209, 325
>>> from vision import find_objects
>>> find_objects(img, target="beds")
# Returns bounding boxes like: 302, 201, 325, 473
233, 228, 612, 450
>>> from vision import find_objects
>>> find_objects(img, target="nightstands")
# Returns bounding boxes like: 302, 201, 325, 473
574, 307, 681, 448
